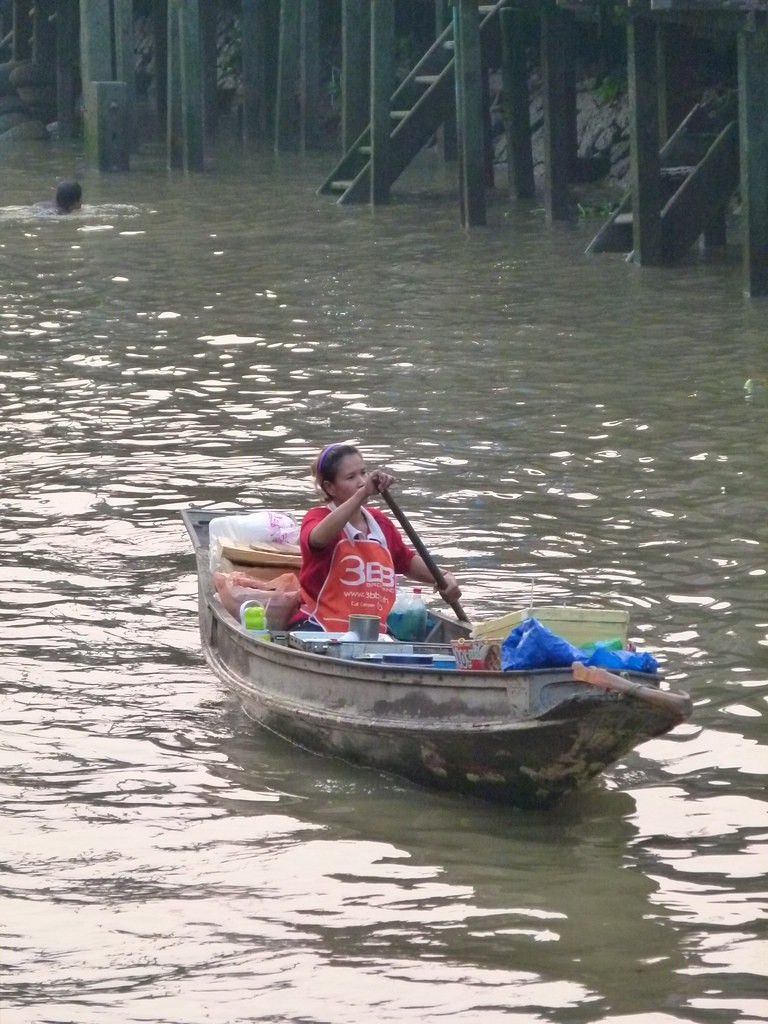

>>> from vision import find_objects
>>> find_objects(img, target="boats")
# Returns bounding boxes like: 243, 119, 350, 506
180, 507, 693, 815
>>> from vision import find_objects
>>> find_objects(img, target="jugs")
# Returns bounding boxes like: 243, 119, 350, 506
239, 599, 271, 642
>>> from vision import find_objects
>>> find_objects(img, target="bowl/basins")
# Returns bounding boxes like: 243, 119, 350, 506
348, 611, 381, 641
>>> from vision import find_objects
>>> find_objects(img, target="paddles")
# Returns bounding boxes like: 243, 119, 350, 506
372, 478, 470, 623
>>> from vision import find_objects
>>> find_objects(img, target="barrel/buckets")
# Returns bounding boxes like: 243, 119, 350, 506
451, 637, 503, 670
352, 652, 457, 670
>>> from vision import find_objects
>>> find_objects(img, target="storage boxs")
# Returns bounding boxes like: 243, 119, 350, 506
473, 607, 630, 651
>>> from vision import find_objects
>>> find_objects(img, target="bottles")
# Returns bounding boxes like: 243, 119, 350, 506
208, 511, 301, 573
578, 637, 622, 657
401, 587, 429, 642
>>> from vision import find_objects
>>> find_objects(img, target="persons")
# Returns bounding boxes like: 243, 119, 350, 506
55, 180, 82, 216
287, 442, 462, 632
